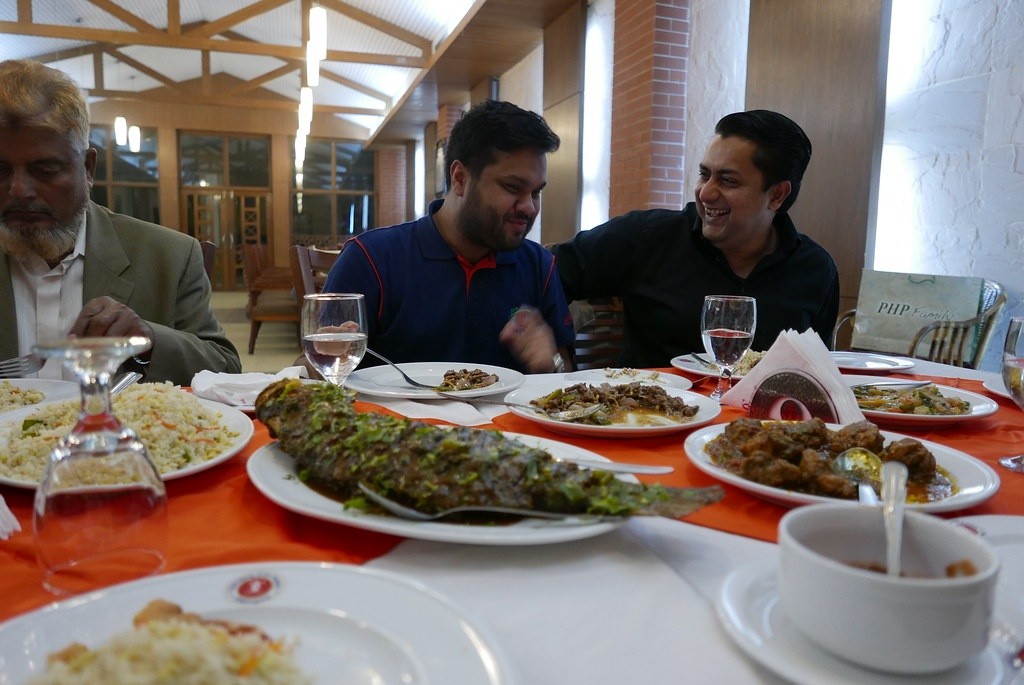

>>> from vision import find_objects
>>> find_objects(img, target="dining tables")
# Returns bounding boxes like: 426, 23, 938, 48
0, 367, 1024, 685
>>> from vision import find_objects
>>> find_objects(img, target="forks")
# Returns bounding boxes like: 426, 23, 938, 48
0, 353, 43, 379
366, 347, 438, 389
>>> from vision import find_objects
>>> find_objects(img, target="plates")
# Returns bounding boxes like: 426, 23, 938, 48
0, 559, 511, 685
829, 351, 916, 372
565, 368, 694, 390
843, 373, 1001, 427
503, 380, 723, 437
252, 429, 641, 546
0, 388, 254, 489
670, 352, 747, 379
714, 551, 1023, 685
684, 419, 1002, 513
344, 361, 526, 400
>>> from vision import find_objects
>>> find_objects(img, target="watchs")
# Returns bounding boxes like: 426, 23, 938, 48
553, 352, 566, 373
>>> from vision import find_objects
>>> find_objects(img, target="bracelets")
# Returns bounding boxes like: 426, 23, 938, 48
133, 355, 150, 364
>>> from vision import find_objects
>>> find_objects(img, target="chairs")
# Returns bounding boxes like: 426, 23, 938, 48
831, 279, 1007, 370
239, 243, 344, 355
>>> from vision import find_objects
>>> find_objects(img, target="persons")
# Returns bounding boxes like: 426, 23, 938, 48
305, 101, 578, 373
0, 60, 242, 387
546, 109, 840, 369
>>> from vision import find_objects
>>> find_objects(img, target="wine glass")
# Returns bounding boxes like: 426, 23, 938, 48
997, 316, 1023, 475
701, 294, 757, 404
28, 337, 172, 596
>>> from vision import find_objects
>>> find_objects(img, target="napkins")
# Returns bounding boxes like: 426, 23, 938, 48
356, 390, 511, 426
356, 518, 780, 685
718, 327, 867, 422
186, 367, 314, 401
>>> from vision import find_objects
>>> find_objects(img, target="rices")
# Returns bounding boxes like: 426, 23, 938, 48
28, 619, 313, 685
1, 378, 242, 489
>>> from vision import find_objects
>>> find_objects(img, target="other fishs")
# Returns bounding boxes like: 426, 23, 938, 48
249, 375, 722, 524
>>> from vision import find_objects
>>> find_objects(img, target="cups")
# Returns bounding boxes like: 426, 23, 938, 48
300, 292, 369, 388
775, 500, 1001, 673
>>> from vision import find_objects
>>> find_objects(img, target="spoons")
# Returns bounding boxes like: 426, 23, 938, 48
356, 480, 575, 522
